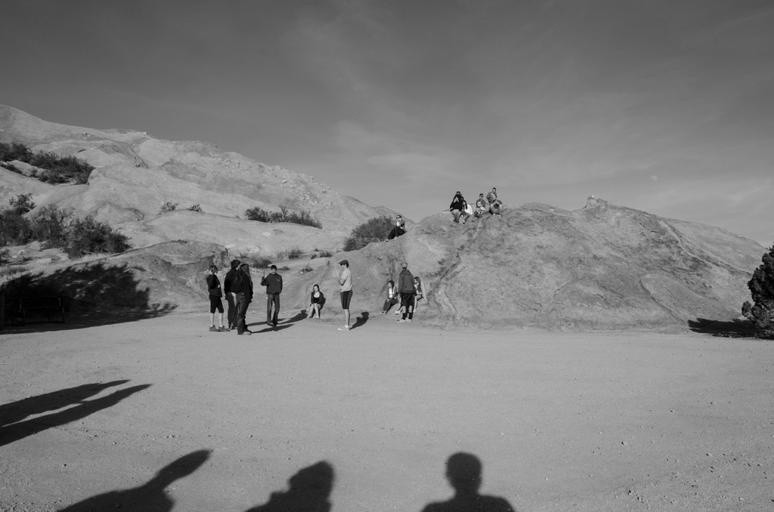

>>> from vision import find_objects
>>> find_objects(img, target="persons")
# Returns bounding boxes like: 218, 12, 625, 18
258, 265, 282, 326
206, 264, 228, 331
306, 283, 323, 318
335, 260, 353, 331
233, 264, 254, 334
379, 280, 398, 315
384, 214, 405, 242
222, 260, 240, 330
397, 262, 416, 323
450, 191, 469, 222
473, 192, 487, 218
487, 187, 501, 215
394, 276, 422, 312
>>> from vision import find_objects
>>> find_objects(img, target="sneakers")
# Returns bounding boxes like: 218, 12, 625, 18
265, 321, 277, 329
379, 311, 412, 321
209, 325, 252, 335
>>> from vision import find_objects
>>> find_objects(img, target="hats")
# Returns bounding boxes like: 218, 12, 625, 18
337, 259, 348, 264
